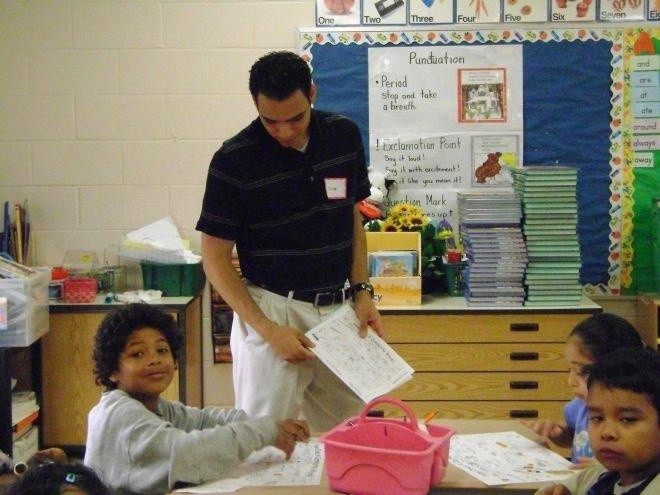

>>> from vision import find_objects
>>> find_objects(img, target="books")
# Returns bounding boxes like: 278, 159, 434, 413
454, 162, 584, 305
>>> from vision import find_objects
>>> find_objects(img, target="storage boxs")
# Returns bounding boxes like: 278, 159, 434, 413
0, 266, 52, 348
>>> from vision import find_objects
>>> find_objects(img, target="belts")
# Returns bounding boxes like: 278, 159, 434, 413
254, 280, 354, 309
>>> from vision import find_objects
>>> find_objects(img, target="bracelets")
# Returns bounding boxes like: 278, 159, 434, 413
350, 282, 374, 302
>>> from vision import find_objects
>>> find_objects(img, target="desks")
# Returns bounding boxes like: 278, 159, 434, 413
156, 417, 568, 495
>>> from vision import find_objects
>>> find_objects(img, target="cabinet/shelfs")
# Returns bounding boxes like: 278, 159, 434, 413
375, 292, 602, 417
0, 337, 43, 474
42, 286, 203, 453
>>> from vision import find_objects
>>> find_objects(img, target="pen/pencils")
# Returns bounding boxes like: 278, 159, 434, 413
424, 409, 439, 424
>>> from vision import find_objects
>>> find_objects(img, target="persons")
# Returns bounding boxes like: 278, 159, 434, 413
6, 462, 114, 495
85, 298, 312, 493
194, 47, 396, 433
517, 305, 645, 466
532, 347, 645, 495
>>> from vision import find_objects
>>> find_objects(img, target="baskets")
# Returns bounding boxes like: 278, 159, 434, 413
62, 276, 97, 303
